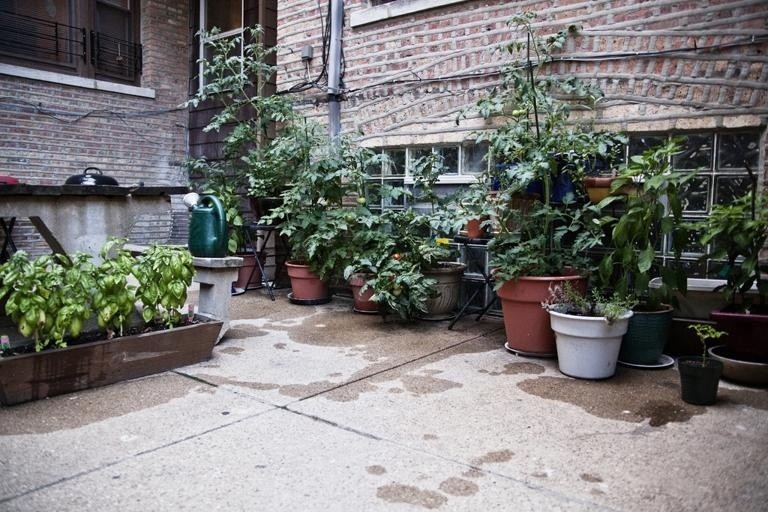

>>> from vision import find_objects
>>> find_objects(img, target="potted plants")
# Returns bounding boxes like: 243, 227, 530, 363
0, 234, 222, 405
177, 11, 768, 406
